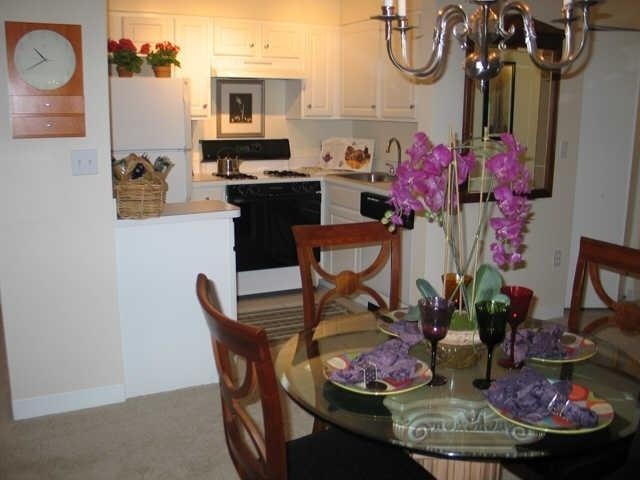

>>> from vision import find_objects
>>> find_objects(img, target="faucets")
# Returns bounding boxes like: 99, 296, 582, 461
385, 137, 402, 176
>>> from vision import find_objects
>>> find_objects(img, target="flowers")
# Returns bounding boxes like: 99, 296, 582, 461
139, 41, 184, 66
379, 133, 534, 310
107, 38, 144, 74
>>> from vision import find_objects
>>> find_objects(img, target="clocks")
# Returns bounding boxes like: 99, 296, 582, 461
4, 20, 86, 97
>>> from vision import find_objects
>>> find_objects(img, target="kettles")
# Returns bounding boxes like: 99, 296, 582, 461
216, 146, 239, 175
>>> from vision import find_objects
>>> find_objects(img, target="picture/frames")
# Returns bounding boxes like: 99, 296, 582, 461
215, 80, 266, 138
483, 61, 516, 142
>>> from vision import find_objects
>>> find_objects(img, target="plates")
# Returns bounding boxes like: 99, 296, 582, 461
377, 325, 399, 337
531, 328, 598, 363
487, 383, 614, 434
322, 347, 433, 395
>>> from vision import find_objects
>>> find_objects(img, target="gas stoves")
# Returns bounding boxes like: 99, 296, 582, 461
212, 170, 310, 180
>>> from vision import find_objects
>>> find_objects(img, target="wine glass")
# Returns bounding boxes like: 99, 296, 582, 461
473, 300, 510, 390
418, 295, 458, 386
497, 286, 533, 369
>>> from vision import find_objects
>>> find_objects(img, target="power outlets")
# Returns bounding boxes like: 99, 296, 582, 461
553, 249, 561, 267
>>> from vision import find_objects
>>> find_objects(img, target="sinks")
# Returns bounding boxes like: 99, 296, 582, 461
328, 171, 399, 185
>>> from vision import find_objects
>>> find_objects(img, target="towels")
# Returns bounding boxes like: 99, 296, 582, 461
391, 306, 423, 350
336, 331, 421, 391
509, 329, 561, 369
488, 376, 597, 433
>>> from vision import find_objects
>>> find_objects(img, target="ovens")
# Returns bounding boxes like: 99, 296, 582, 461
225, 181, 323, 275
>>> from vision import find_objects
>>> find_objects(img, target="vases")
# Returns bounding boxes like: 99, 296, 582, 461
153, 65, 171, 77
118, 65, 133, 78
419, 313, 484, 345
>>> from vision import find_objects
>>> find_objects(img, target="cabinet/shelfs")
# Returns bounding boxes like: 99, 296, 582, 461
108, 8, 213, 120
381, 10, 417, 122
214, 19, 307, 80
343, 20, 381, 120
326, 186, 425, 325
304, 24, 341, 119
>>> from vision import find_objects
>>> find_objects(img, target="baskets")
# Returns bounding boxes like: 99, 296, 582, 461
113, 155, 168, 218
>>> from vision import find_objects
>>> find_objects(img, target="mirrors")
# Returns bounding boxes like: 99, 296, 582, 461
456, 13, 565, 202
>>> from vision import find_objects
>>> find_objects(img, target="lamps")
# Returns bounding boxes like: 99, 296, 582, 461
373, 1, 590, 79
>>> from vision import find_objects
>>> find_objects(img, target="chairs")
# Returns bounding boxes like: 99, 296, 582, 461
499, 237, 640, 480
196, 274, 440, 480
292, 215, 401, 437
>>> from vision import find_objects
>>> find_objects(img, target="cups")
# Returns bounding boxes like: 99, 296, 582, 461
441, 272, 472, 311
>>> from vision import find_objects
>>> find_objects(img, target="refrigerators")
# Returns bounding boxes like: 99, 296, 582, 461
111, 78, 190, 203
